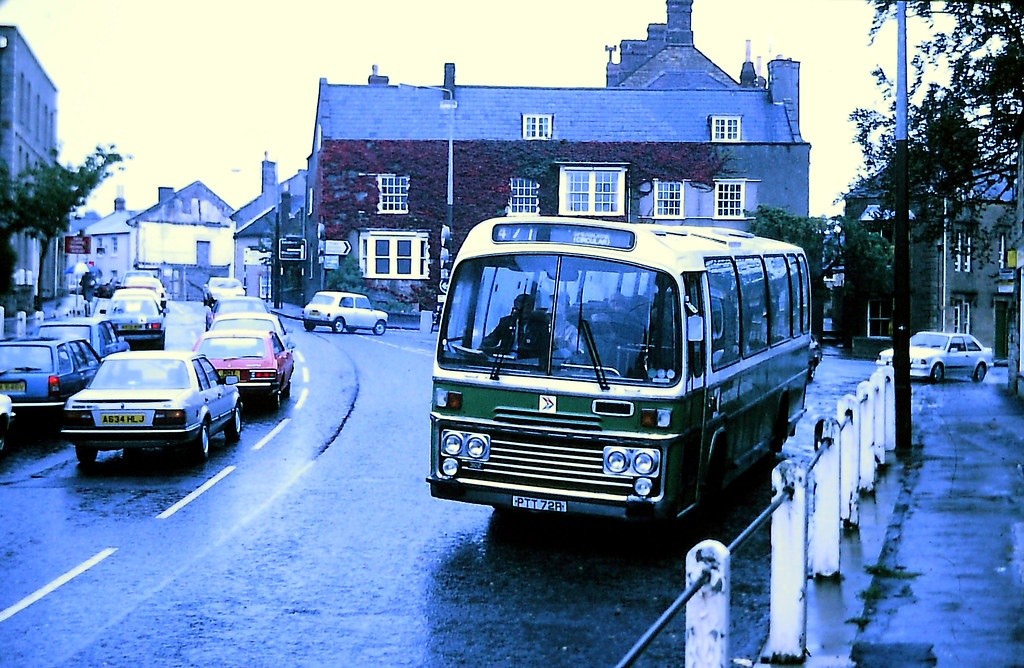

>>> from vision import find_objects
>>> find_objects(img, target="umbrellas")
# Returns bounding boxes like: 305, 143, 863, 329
60, 262, 102, 275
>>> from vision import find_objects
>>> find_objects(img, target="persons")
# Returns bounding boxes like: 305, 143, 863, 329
79, 272, 98, 304
478, 293, 632, 360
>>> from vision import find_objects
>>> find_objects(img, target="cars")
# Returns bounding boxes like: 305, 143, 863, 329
0, 392, 16, 452
203, 276, 248, 306
302, 290, 389, 336
100, 286, 169, 348
807, 334, 822, 383
205, 296, 273, 334
192, 329, 296, 409
116, 270, 155, 290
876, 331, 994, 386
61, 349, 245, 465
209, 312, 297, 356
117, 276, 170, 317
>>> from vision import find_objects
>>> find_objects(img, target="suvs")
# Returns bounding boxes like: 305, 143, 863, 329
0, 338, 104, 431
35, 317, 130, 363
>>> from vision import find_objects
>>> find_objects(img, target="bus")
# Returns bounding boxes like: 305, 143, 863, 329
424, 212, 813, 526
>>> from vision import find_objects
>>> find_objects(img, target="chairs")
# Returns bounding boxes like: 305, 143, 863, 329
555, 275, 811, 378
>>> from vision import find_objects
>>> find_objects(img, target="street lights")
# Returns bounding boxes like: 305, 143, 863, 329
231, 166, 285, 310
386, 74, 453, 332
